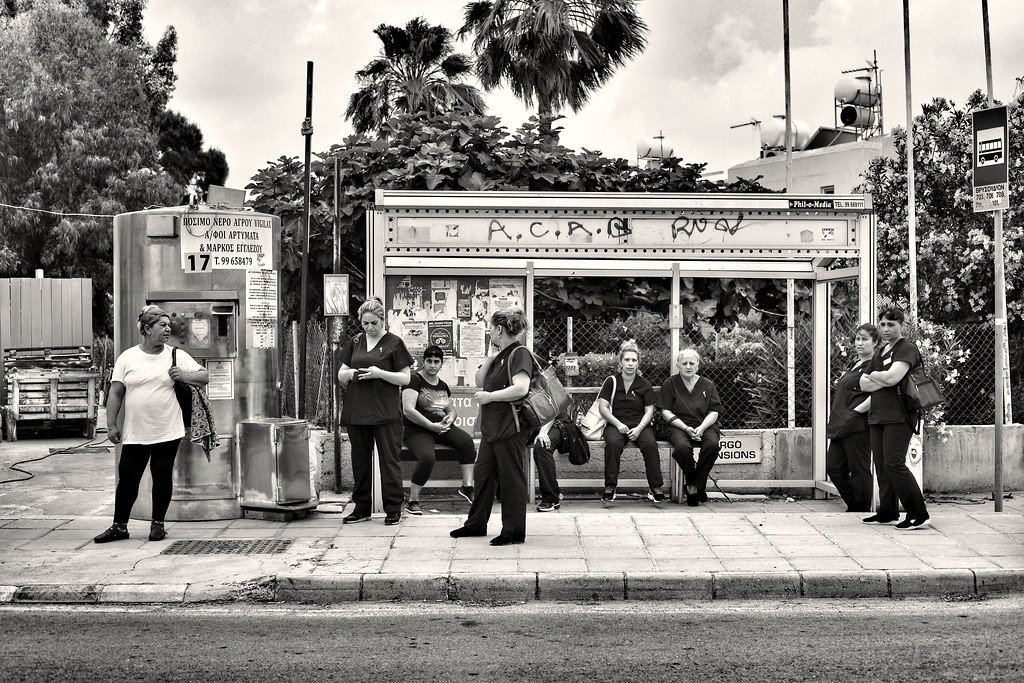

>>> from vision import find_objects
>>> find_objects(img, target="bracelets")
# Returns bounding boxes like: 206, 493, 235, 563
685, 427, 688, 431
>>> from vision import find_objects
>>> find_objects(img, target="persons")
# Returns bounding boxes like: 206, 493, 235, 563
827, 324, 882, 512
338, 297, 416, 525
657, 349, 721, 506
533, 413, 568, 511
599, 347, 666, 503
402, 346, 476, 514
450, 311, 533, 545
94, 309, 210, 542
860, 308, 931, 530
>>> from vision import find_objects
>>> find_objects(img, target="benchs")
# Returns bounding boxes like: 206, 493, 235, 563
374, 388, 684, 500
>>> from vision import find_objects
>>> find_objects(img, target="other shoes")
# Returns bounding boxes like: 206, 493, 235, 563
697, 488, 708, 503
683, 483, 699, 506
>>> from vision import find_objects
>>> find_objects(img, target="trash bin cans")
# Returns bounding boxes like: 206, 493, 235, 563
241, 418, 311, 505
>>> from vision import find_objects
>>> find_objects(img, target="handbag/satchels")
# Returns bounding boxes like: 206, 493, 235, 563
171, 346, 192, 427
579, 375, 617, 441
567, 423, 590, 465
653, 412, 679, 441
558, 424, 569, 454
508, 346, 573, 429
891, 341, 944, 412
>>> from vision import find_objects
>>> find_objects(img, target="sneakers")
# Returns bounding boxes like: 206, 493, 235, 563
94, 525, 130, 543
601, 485, 617, 502
149, 521, 167, 540
343, 509, 371, 524
405, 501, 423, 515
537, 501, 560, 512
490, 535, 524, 546
458, 487, 474, 504
450, 526, 487, 537
895, 514, 931, 530
648, 486, 667, 503
384, 512, 402, 525
862, 513, 899, 525
